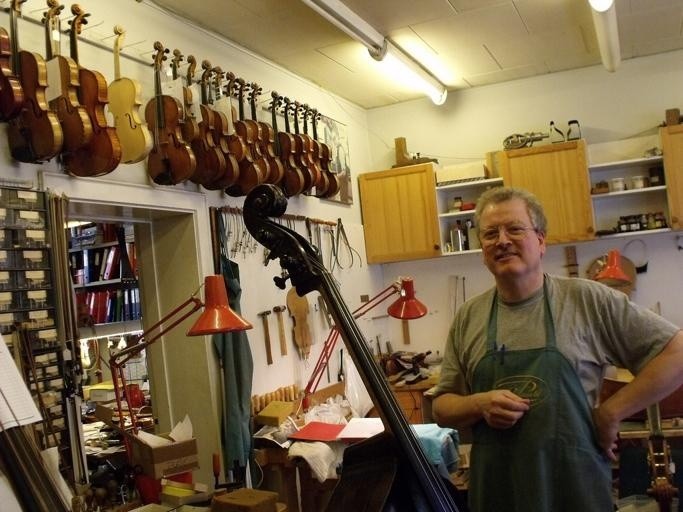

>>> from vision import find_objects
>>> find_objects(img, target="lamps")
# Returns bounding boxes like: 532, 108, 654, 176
375, 41, 447, 106
108, 275, 254, 504
586, 1, 624, 77
288, 275, 431, 426
303, 1, 386, 58
593, 249, 634, 290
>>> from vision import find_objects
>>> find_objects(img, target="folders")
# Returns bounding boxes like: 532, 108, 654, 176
81, 287, 142, 323
80, 246, 120, 283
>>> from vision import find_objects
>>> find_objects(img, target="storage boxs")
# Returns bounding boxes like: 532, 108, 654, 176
122, 424, 201, 476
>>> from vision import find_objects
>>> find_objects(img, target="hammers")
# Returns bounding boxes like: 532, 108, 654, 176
257, 311, 274, 365
273, 305, 288, 357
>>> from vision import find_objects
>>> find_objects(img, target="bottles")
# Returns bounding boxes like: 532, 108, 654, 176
665, 445, 675, 480
452, 196, 462, 207
618, 210, 666, 232
444, 217, 467, 252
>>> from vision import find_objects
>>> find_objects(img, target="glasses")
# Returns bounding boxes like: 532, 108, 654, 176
481, 224, 536, 246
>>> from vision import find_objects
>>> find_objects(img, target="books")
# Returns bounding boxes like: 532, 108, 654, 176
67, 222, 141, 326
289, 421, 346, 442
337, 418, 389, 439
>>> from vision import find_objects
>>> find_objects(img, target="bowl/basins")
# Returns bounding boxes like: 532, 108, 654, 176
461, 203, 475, 210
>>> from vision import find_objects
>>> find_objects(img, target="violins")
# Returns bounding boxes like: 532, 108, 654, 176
169, 48, 200, 143
59, 3, 122, 177
110, 24, 155, 167
145, 40, 198, 186
41, 0, 94, 159
200, 59, 240, 190
206, 65, 329, 198
6, 0, 64, 165
310, 107, 339, 199
0, 26, 25, 122
184, 54, 227, 182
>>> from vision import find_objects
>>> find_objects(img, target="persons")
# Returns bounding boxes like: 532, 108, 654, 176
431, 186, 682, 512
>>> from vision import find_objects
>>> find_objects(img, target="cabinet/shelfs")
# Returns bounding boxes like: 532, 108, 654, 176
358, 162, 444, 265
585, 156, 669, 241
502, 139, 598, 245
64, 223, 144, 344
366, 372, 429, 427
436, 176, 504, 252
659, 123, 683, 233
